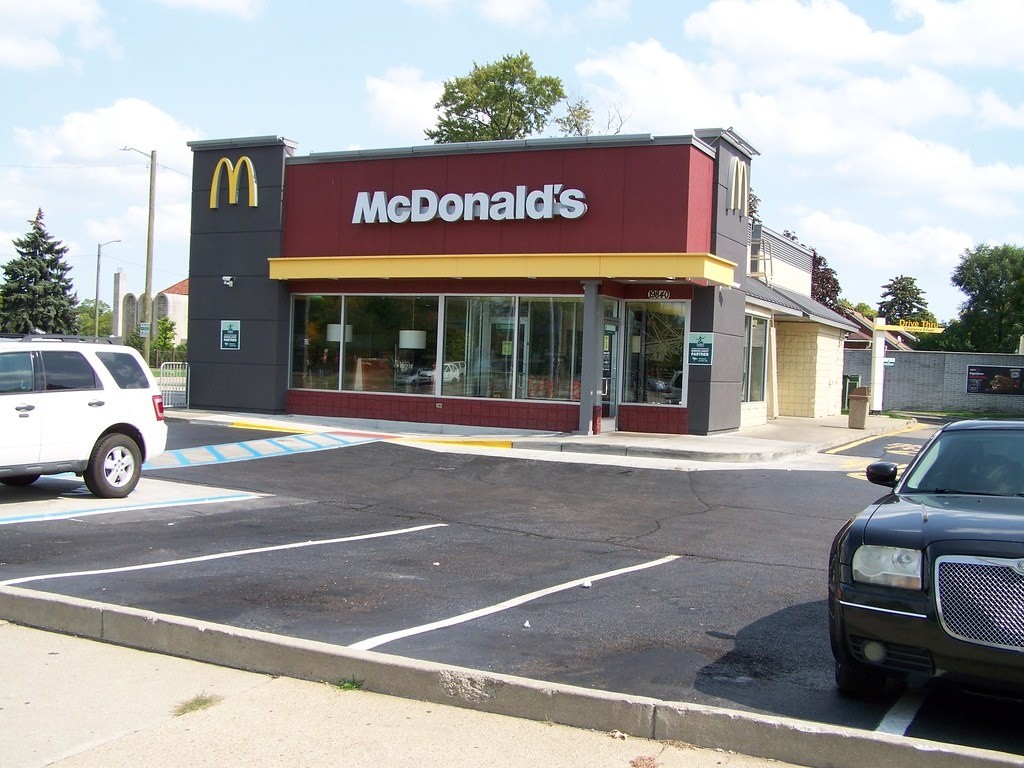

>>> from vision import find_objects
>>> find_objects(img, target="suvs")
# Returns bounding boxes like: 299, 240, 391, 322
0, 332, 169, 499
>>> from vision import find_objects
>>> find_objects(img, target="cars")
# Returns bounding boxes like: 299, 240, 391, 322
602, 353, 612, 377
393, 358, 465, 387
824, 420, 1023, 703
647, 371, 683, 404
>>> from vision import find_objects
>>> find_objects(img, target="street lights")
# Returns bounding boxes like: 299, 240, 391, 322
120, 146, 157, 368
94, 239, 122, 342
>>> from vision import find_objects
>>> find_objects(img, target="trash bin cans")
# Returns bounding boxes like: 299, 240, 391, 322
848, 386, 871, 428
